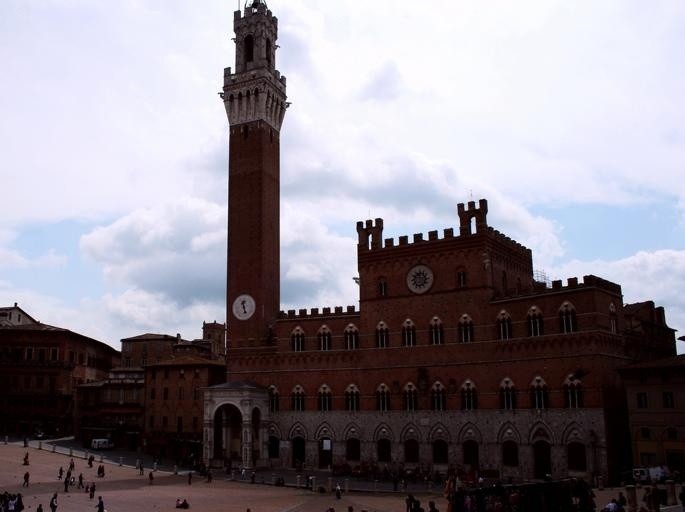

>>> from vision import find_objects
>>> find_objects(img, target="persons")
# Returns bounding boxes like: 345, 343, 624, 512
660, 465, 665, 484
274, 477, 284, 487
405, 462, 463, 512
238, 464, 257, 484
175, 499, 188, 508
207, 470, 212, 483
599, 484, 662, 512
335, 484, 341, 499
678, 485, 685, 512
0, 451, 160, 512
188, 472, 192, 485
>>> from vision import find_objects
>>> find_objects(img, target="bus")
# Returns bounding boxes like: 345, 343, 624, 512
452, 479, 597, 511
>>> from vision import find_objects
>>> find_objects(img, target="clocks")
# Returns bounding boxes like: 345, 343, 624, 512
232, 294, 256, 321
